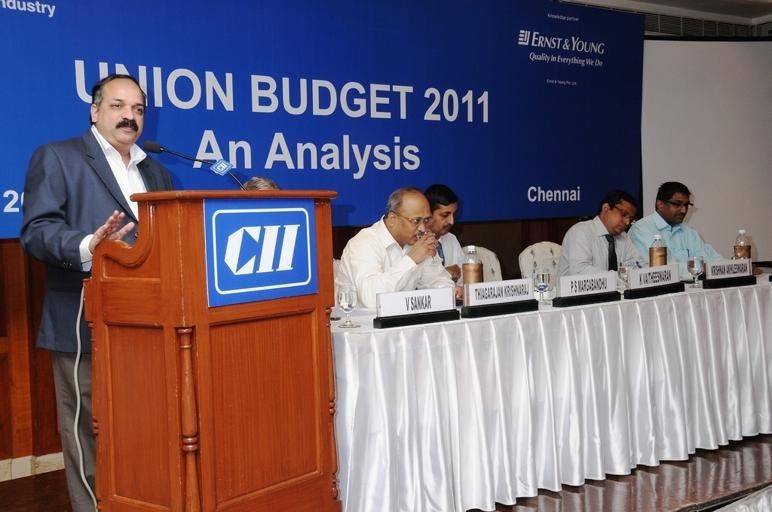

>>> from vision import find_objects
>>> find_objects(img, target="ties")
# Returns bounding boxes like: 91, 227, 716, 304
604, 235, 617, 271
436, 242, 445, 265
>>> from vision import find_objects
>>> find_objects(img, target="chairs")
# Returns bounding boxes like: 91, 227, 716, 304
333, 260, 352, 303
462, 245, 502, 285
517, 241, 563, 287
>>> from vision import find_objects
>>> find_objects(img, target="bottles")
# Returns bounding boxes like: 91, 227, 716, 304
649, 235, 667, 266
463, 244, 483, 282
734, 229, 751, 259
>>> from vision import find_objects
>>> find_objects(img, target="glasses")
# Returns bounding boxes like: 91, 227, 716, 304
664, 200, 694, 208
612, 205, 638, 223
389, 209, 430, 226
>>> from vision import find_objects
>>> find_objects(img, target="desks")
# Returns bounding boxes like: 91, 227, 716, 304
331, 271, 772, 512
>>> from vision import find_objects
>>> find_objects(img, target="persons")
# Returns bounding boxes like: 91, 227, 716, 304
243, 176, 279, 191
334, 186, 455, 310
19, 73, 171, 512
403, 185, 469, 301
555, 188, 647, 289
627, 180, 763, 284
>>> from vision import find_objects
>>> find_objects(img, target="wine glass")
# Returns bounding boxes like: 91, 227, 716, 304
533, 273, 551, 305
616, 274, 630, 300
687, 256, 704, 291
417, 232, 445, 265
337, 291, 359, 328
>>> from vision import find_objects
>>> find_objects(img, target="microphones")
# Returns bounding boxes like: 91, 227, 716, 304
143, 141, 249, 192
